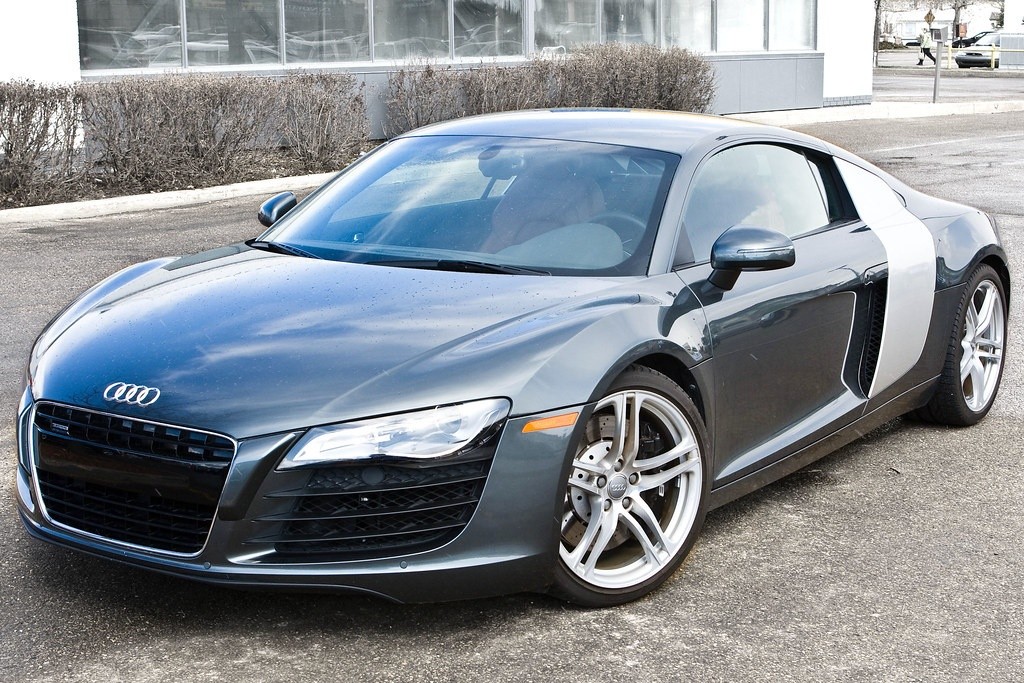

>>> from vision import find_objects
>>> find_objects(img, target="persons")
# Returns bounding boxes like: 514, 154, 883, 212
916, 28, 936, 67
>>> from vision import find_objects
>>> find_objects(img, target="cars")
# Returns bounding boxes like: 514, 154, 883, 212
952, 30, 997, 48
80, 23, 656, 68
954, 31, 1000, 68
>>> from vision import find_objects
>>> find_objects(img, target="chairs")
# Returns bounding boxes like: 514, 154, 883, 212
681, 173, 779, 261
479, 171, 605, 253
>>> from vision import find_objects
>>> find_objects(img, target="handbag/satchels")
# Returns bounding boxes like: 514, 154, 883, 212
917, 47, 925, 61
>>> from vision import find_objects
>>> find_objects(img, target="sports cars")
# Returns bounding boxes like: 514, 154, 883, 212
10, 104, 1013, 611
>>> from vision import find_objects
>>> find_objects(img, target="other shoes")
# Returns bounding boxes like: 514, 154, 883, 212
917, 62, 923, 65
934, 59, 936, 65
